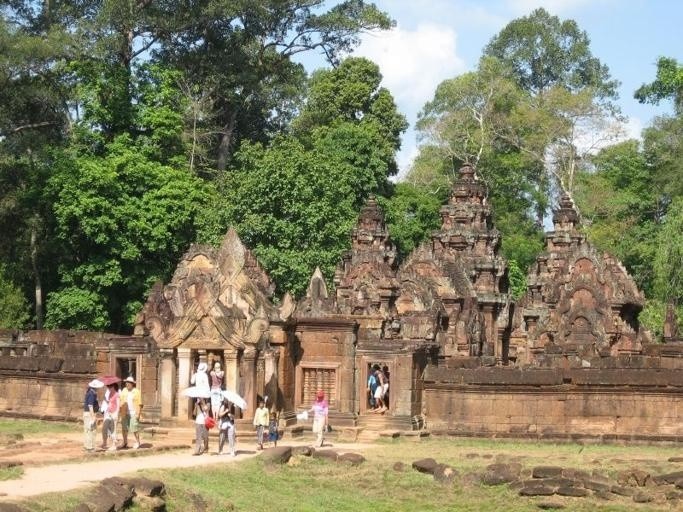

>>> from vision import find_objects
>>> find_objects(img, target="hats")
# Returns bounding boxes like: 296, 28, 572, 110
87, 379, 105, 389
196, 362, 209, 373
214, 362, 221, 373
123, 377, 137, 386
315, 391, 325, 398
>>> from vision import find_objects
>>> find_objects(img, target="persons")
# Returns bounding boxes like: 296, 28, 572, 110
116, 376, 144, 450
366, 366, 378, 411
215, 397, 236, 458
191, 398, 210, 457
252, 400, 269, 451
96, 383, 121, 452
267, 411, 279, 448
189, 362, 210, 421
208, 361, 225, 422
381, 365, 388, 403
303, 390, 329, 449
82, 379, 105, 451
100, 388, 121, 443
371, 364, 389, 414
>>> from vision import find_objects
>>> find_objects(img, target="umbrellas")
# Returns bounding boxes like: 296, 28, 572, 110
180, 385, 212, 401
95, 374, 122, 396
213, 389, 247, 411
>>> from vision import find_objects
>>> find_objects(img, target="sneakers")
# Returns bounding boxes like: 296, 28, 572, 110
193, 447, 237, 459
80, 443, 143, 454
